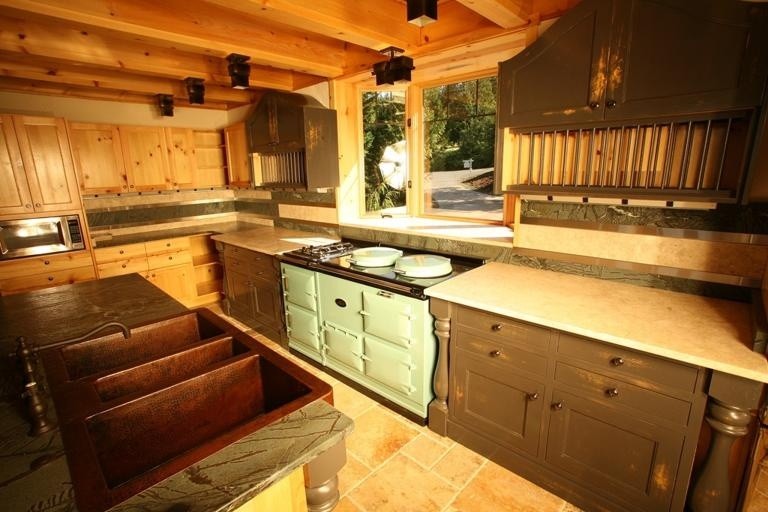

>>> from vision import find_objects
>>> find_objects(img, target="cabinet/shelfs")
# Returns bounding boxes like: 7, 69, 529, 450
194, 130, 230, 189
226, 122, 254, 182
248, 108, 338, 190
189, 231, 224, 296
450, 306, 702, 509
222, 245, 278, 334
168, 126, 195, 190
1, 243, 94, 298
1, 115, 83, 219
491, 1, 767, 206
69, 126, 169, 199
96, 236, 196, 309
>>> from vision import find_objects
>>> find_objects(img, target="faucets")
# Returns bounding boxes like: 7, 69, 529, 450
33, 321, 131, 354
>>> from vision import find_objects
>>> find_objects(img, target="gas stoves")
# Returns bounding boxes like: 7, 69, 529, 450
283, 241, 357, 263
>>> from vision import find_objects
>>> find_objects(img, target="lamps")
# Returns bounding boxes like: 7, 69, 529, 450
154, 53, 251, 118
373, 0, 437, 91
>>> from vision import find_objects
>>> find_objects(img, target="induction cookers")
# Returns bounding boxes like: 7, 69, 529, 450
316, 245, 482, 290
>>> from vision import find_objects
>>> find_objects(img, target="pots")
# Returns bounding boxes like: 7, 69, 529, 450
391, 251, 453, 278
345, 243, 403, 268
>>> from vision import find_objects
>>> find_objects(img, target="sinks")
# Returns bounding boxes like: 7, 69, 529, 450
92, 334, 258, 404
60, 307, 230, 383
81, 352, 314, 491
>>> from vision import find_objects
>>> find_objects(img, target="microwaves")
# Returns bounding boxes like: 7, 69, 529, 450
1, 214, 86, 262
